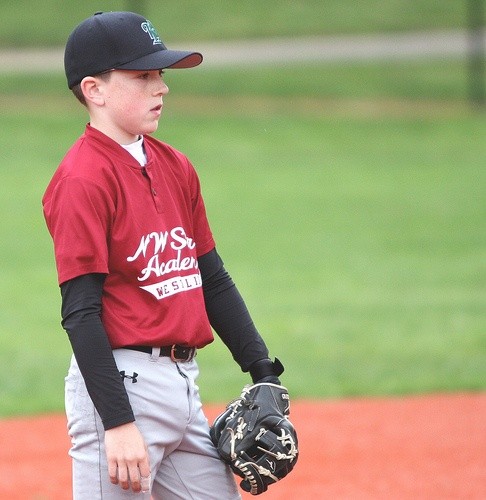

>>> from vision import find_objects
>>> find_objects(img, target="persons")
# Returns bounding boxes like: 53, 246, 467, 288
40, 11, 292, 499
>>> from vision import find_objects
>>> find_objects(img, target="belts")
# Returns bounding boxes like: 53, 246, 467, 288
121, 344, 197, 362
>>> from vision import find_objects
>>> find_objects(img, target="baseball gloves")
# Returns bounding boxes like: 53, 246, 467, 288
210, 381, 299, 497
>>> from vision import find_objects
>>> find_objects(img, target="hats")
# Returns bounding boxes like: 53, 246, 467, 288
64, 10, 203, 90
